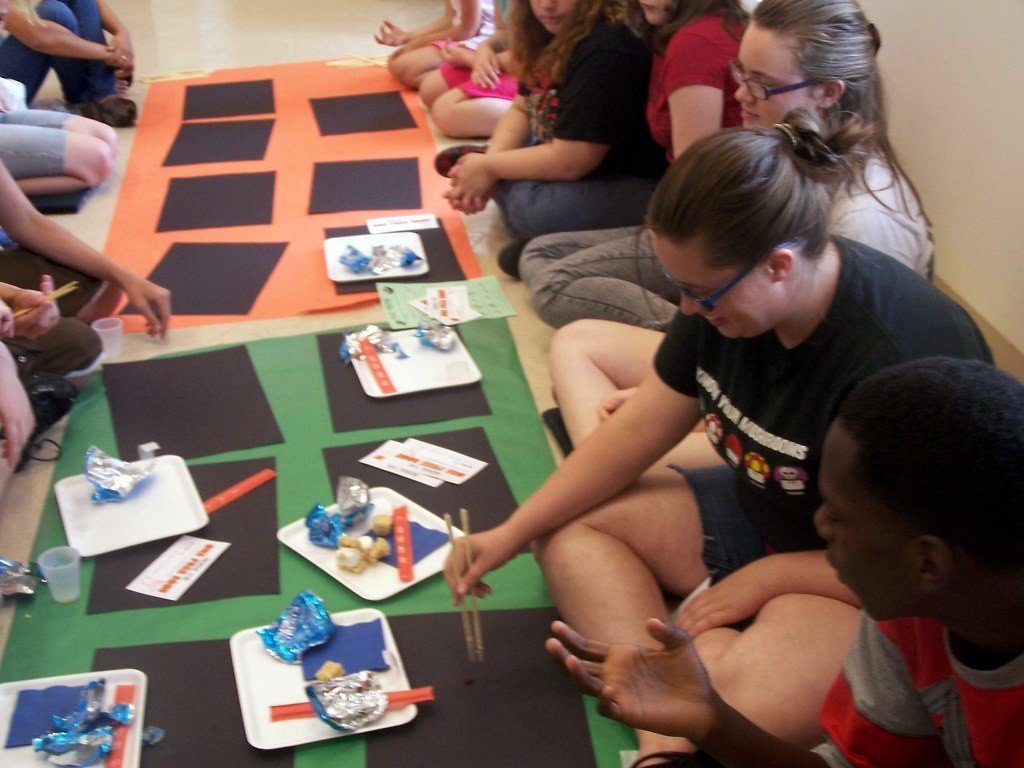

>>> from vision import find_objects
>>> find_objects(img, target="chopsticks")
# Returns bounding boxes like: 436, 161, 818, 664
443, 510, 486, 663
7, 279, 81, 319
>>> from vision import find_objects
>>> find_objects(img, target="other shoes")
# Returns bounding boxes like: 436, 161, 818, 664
434, 143, 488, 178
630, 748, 722, 768
66, 96, 136, 126
497, 242, 523, 279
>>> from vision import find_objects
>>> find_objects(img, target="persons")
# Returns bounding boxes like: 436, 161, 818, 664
421, 0, 520, 137
445, 108, 996, 768
0, 342, 35, 469
519, 0, 750, 331
549, 355, 1024, 768
548, 0, 934, 473
0, 0, 138, 128
434, 0, 669, 278
372, 0, 495, 90
0, 78, 119, 195
0, 159, 171, 376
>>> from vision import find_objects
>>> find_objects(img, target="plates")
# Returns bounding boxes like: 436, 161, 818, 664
228, 609, 419, 751
55, 454, 209, 557
322, 230, 430, 282
346, 327, 483, 399
1, 668, 148, 768
276, 487, 474, 603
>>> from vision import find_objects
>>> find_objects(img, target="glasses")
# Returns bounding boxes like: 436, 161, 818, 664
661, 237, 800, 312
729, 60, 823, 100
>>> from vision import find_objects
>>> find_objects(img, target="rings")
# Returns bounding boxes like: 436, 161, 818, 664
121, 55, 127, 60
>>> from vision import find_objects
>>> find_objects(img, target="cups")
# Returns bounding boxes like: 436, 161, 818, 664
37, 546, 80, 603
91, 316, 124, 358
